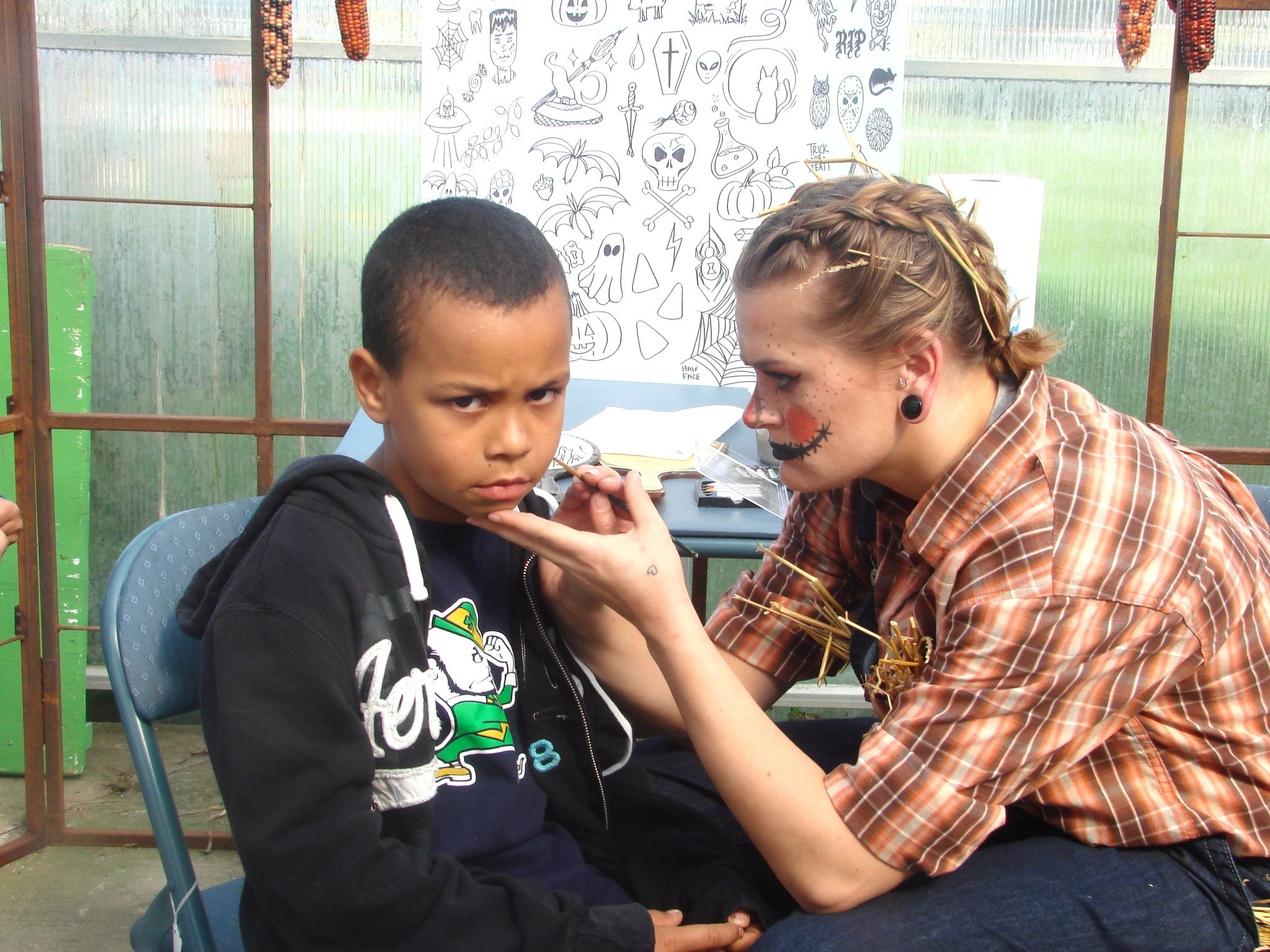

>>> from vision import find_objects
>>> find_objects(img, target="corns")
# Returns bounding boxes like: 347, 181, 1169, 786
260, 0, 293, 89
1179, 0, 1216, 72
335, 0, 370, 61
1116, 0, 1156, 72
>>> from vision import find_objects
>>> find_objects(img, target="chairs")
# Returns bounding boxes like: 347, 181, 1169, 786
98, 497, 270, 952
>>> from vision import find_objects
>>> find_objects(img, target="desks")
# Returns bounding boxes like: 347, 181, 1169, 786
332, 377, 796, 632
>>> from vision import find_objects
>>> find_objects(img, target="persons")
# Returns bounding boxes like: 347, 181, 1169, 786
171, 192, 763, 950
465, 175, 1270, 952
0, 498, 27, 561
